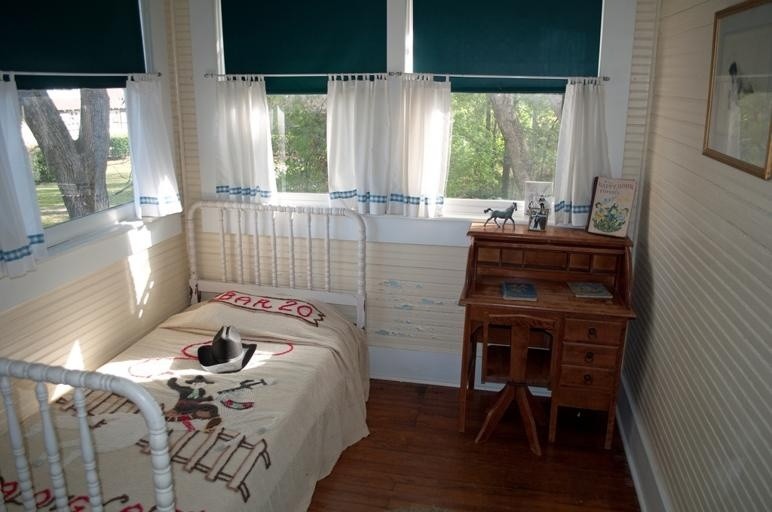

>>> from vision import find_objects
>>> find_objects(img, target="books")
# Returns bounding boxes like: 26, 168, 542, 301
500, 281, 538, 302
587, 176, 636, 239
566, 280, 614, 299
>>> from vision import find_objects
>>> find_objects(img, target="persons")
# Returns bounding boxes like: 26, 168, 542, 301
530, 194, 546, 230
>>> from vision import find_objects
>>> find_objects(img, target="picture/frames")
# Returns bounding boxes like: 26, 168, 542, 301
702, 0, 772, 180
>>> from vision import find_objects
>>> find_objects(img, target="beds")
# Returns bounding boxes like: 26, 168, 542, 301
0, 202, 370, 512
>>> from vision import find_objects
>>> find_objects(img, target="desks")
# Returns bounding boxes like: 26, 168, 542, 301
457, 223, 636, 450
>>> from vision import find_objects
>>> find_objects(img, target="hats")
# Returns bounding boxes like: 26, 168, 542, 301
197, 324, 257, 375
184, 375, 215, 384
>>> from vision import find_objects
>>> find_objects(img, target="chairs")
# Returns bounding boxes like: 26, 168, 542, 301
474, 312, 559, 456
